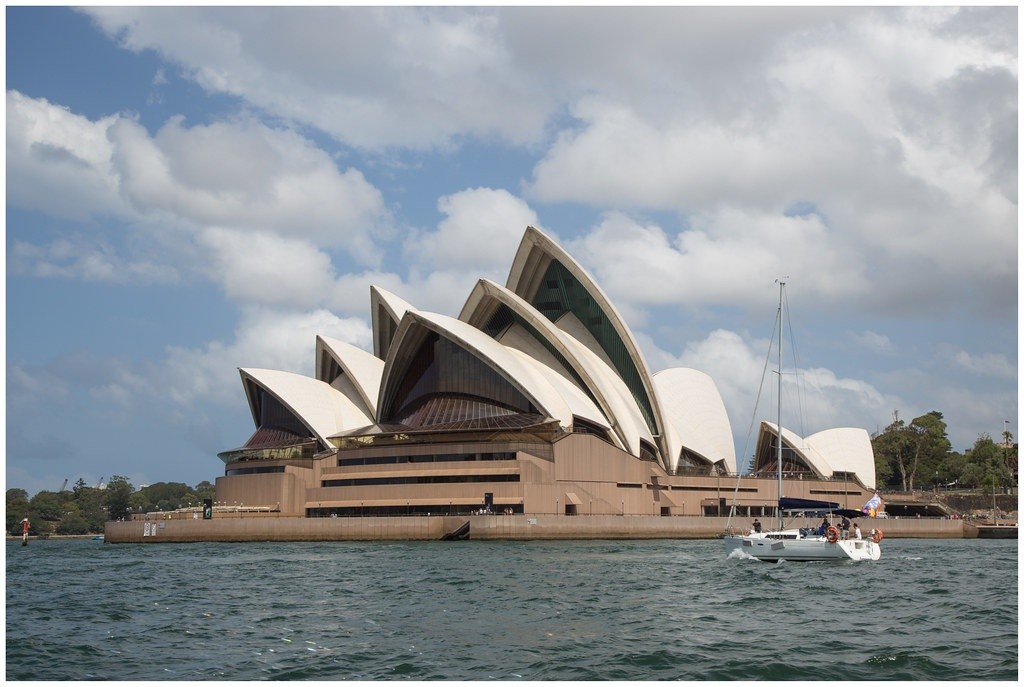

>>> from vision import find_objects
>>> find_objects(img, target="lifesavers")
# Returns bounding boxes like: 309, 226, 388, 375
873, 528, 882, 543
825, 526, 839, 543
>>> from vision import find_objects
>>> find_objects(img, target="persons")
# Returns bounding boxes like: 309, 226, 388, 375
853, 523, 862, 541
837, 517, 851, 540
819, 519, 830, 536
752, 519, 761, 533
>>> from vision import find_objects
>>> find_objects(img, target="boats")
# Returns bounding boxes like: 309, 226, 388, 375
93, 535, 104, 540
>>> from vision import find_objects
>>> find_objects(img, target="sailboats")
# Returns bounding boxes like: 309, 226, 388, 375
722, 274, 883, 562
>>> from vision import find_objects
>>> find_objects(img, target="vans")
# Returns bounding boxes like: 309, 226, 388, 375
876, 512, 889, 519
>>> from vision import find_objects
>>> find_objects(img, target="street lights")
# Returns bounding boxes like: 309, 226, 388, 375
481, 499, 484, 514
155, 506, 159, 519
126, 507, 129, 521
622, 500, 624, 514
520, 499, 523, 512
652, 500, 656, 514
234, 501, 237, 518
904, 505, 907, 518
277, 502, 279, 518
925, 506, 928, 517
318, 501, 321, 518
138, 506, 142, 521
590, 500, 592, 515
130, 506, 132, 519
556, 499, 559, 514
407, 500, 409, 514
1003, 420, 1010, 445
450, 499, 451, 515
362, 501, 363, 516
682, 501, 684, 515
710, 501, 714, 515
241, 503, 243, 518
